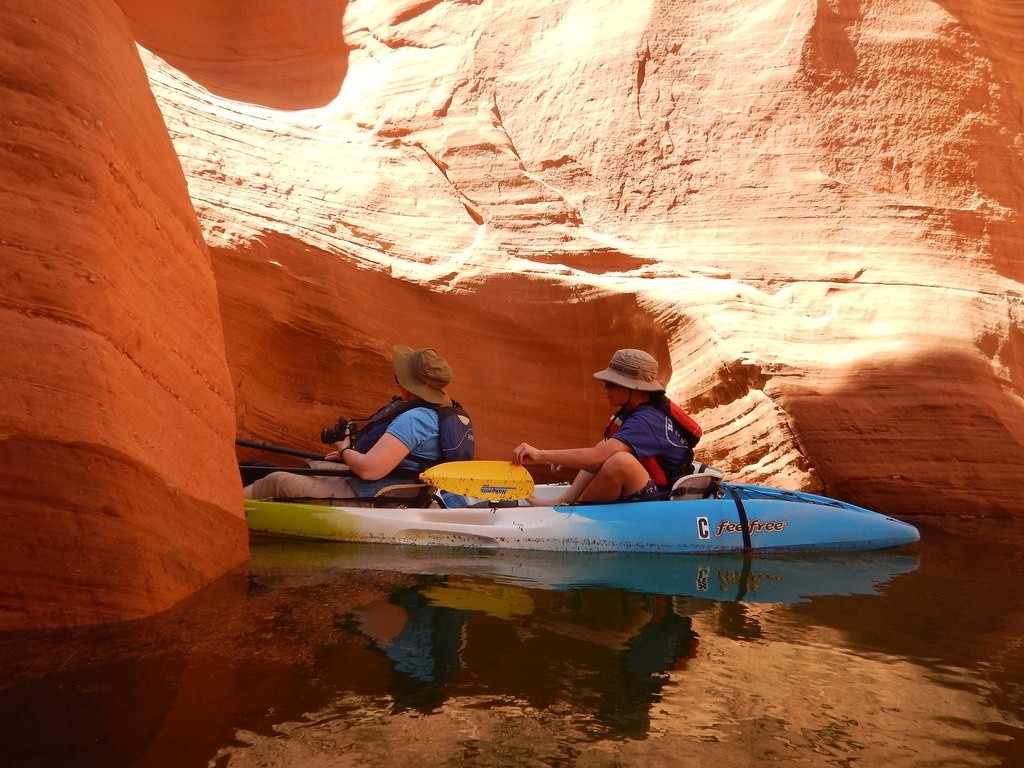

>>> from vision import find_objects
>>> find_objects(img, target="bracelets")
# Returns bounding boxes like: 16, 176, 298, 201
340, 448, 347, 459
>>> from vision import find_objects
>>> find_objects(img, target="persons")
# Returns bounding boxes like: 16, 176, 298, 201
509, 349, 694, 506
243, 344, 453, 508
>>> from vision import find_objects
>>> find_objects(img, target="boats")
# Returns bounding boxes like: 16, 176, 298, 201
245, 480, 922, 554
243, 542, 919, 611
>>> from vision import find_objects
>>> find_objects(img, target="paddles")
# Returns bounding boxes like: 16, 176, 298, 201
233, 438, 536, 502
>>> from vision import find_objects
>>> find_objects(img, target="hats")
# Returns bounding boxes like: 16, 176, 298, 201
392, 345, 452, 404
592, 349, 665, 400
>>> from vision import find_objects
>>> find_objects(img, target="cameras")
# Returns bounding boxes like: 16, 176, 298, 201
321, 416, 357, 450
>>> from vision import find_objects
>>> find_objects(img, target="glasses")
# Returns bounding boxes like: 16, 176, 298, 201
394, 374, 400, 384
606, 382, 617, 389
